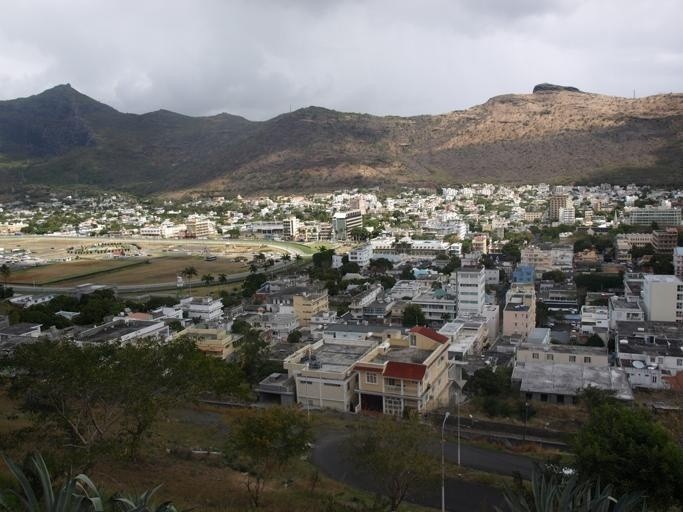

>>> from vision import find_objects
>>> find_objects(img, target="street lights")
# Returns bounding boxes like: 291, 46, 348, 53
440, 412, 451, 512
